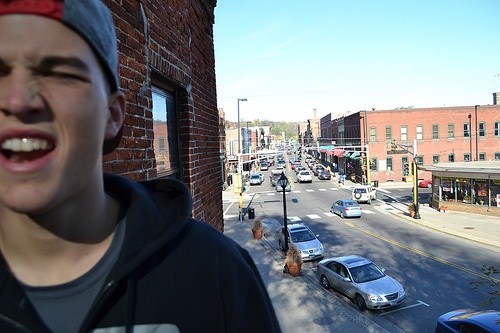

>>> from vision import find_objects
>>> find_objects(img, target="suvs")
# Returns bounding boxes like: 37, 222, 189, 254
351, 185, 377, 205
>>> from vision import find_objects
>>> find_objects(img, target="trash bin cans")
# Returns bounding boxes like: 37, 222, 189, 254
374, 181, 378, 187
248, 209, 254, 219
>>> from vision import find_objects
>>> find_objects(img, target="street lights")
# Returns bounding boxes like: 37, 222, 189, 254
475, 105, 480, 161
279, 170, 288, 256
238, 98, 248, 153
247, 121, 252, 152
468, 113, 472, 161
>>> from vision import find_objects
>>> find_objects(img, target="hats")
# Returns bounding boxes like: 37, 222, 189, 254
0, 0, 125, 156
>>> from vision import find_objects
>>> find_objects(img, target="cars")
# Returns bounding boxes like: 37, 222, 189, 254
275, 180, 291, 192
279, 223, 324, 262
256, 151, 332, 185
318, 255, 406, 311
435, 309, 500, 333
419, 179, 433, 188
250, 175, 261, 185
331, 199, 363, 218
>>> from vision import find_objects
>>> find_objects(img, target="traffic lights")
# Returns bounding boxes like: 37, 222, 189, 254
391, 141, 396, 153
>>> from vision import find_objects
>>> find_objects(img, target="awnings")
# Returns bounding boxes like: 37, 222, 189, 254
319, 145, 361, 160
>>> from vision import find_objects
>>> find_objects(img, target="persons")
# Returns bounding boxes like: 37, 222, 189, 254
0, 0, 281, 333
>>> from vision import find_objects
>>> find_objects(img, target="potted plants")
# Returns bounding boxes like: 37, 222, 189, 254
251, 220, 263, 239
285, 243, 301, 275
409, 203, 415, 218
